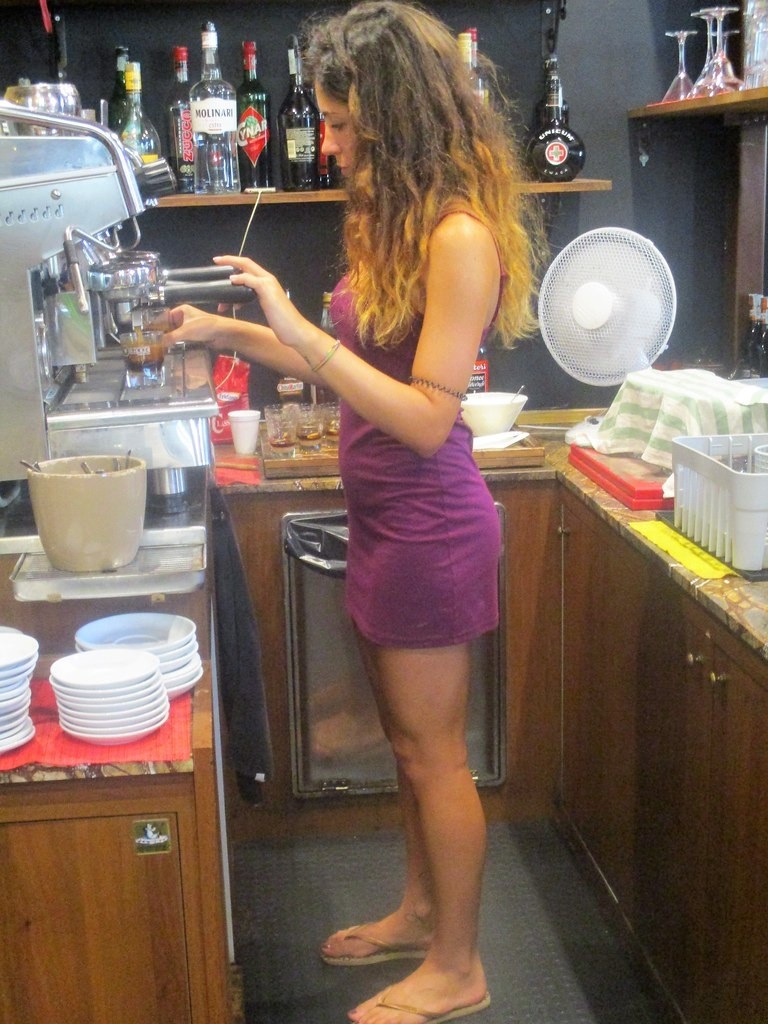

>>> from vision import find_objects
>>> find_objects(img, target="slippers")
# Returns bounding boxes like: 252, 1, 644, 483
320, 921, 427, 967
351, 984, 490, 1024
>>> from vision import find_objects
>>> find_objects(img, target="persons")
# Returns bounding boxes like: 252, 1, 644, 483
139, 0, 550, 1024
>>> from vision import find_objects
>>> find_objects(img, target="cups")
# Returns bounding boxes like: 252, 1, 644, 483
264, 403, 296, 448
754, 445, 768, 475
738, 0, 768, 91
4, 83, 84, 138
295, 403, 323, 440
119, 330, 165, 388
227, 409, 261, 454
146, 306, 171, 336
319, 401, 342, 442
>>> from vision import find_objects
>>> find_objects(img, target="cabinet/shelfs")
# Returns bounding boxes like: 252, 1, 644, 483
1, 420, 768, 1024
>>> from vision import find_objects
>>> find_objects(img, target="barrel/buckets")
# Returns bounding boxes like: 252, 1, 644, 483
26, 454, 147, 573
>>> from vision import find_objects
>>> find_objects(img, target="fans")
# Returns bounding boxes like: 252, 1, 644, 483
536, 227, 677, 445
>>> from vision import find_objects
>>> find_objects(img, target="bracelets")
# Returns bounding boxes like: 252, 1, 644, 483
409, 376, 468, 403
310, 339, 341, 373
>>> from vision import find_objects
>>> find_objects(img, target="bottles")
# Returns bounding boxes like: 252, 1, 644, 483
525, 53, 585, 182
277, 288, 310, 409
465, 345, 489, 395
277, 44, 320, 192
739, 293, 768, 382
165, 46, 197, 194
109, 46, 130, 143
313, 76, 344, 190
188, 21, 241, 195
114, 61, 162, 168
310, 292, 335, 404
456, 26, 495, 115
236, 41, 273, 193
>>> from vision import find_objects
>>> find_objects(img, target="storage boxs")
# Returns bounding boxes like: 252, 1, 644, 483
666, 435, 768, 572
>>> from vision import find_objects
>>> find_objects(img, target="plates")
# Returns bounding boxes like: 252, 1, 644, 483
0, 626, 40, 754
48, 648, 170, 747
73, 612, 204, 702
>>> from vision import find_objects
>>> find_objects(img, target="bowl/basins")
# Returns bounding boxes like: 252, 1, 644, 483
461, 391, 528, 437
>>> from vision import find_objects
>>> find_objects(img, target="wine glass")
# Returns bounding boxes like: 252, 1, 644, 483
686, 6, 745, 101
659, 30, 699, 104
